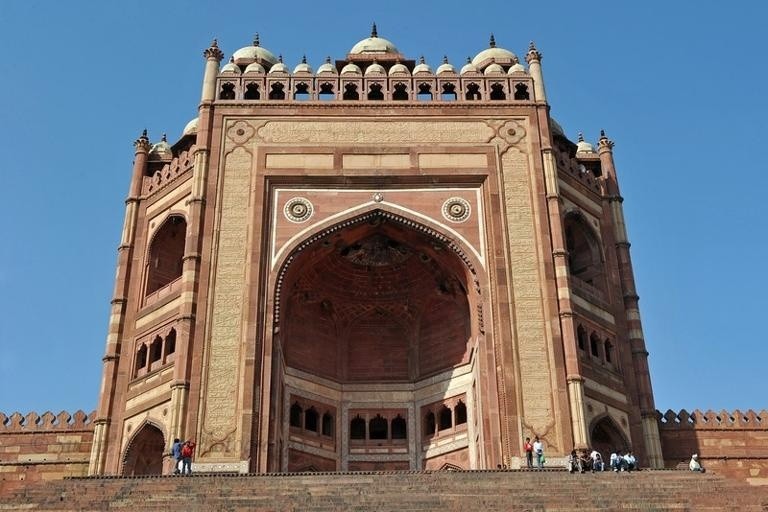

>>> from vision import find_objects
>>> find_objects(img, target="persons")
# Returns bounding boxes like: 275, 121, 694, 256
689, 453, 704, 473
170, 437, 193, 475
568, 447, 640, 473
524, 437, 533, 467
533, 436, 544, 468
181, 440, 196, 474
